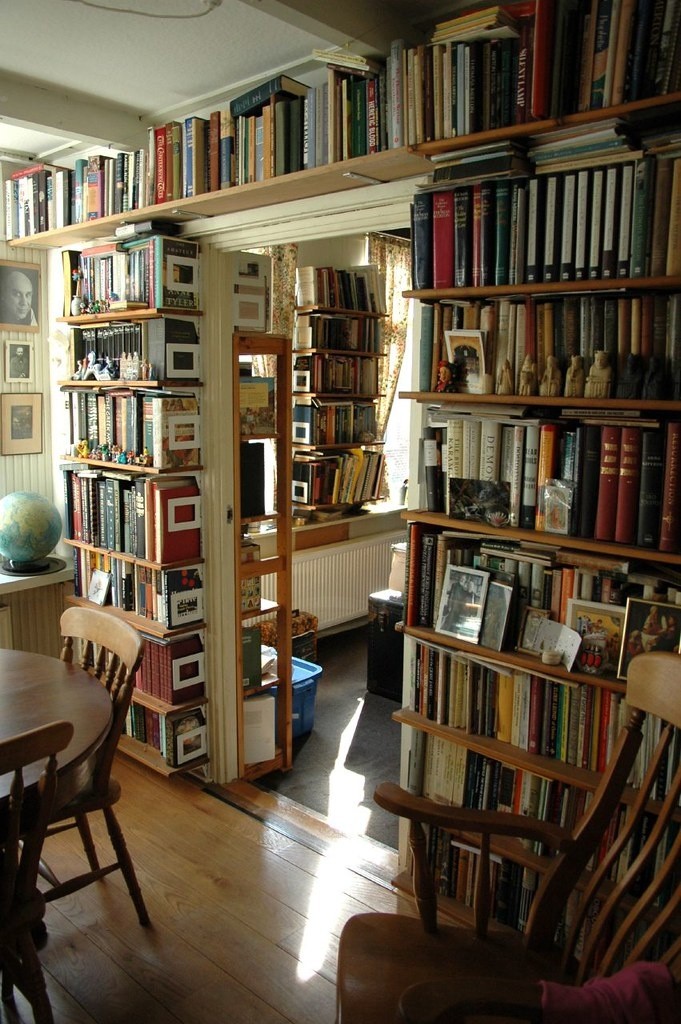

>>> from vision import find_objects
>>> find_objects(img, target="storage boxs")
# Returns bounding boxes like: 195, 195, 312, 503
270, 655, 322, 742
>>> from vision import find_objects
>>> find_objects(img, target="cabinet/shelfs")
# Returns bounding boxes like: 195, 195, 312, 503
8, 93, 681, 937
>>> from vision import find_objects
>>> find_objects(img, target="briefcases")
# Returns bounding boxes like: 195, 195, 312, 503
254, 609, 319, 662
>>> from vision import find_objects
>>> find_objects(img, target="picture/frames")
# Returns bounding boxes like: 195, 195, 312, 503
616, 596, 681, 679
0, 392, 44, 457
0, 258, 42, 334
565, 598, 626, 677
434, 564, 490, 646
516, 606, 552, 658
5, 340, 35, 383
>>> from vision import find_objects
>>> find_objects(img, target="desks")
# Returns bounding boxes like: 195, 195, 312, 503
0, 647, 113, 949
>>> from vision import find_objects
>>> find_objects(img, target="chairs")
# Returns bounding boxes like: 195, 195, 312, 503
17, 607, 151, 929
0, 720, 76, 1024
336, 653, 681, 1024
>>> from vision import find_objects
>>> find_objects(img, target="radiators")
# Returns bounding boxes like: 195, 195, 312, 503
264, 528, 408, 630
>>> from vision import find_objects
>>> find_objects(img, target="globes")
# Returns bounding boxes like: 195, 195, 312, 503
0, 492, 66, 577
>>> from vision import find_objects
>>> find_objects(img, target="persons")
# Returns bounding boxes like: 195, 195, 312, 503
499, 349, 681, 400
464, 581, 479, 617
443, 575, 466, 632
0, 271, 38, 326
433, 360, 460, 392
11, 347, 29, 378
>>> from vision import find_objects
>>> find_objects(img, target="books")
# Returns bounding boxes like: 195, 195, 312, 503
6, 0, 681, 959
